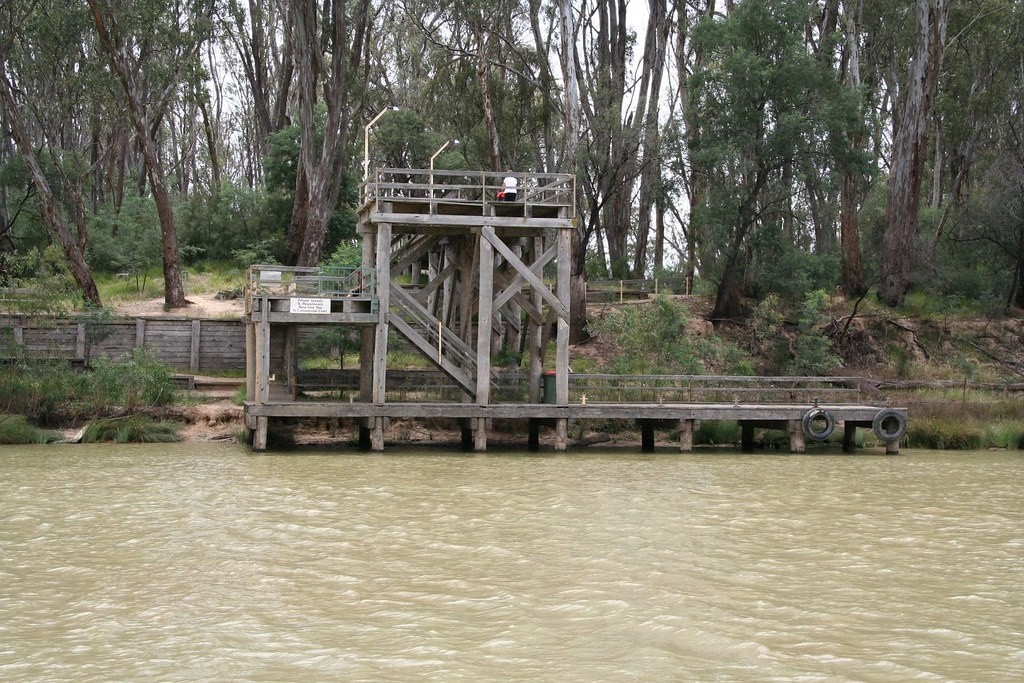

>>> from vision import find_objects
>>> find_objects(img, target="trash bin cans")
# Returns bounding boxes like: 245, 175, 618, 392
255, 272, 282, 294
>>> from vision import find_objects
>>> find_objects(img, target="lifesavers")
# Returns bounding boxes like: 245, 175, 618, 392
797, 406, 835, 440
872, 410, 908, 441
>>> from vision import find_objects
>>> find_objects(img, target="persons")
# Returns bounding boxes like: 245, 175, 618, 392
501, 170, 518, 201
347, 271, 363, 296
